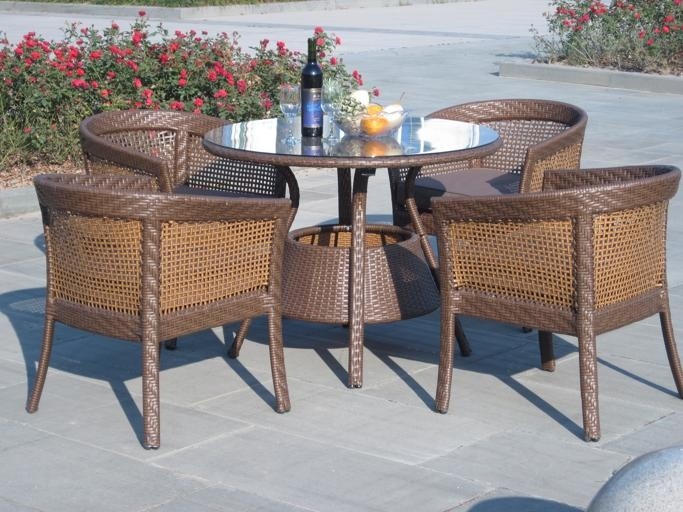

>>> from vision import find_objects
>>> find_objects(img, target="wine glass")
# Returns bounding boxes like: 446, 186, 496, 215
278, 85, 299, 143
315, 84, 343, 139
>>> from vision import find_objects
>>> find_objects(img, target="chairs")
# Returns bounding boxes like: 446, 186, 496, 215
389, 99, 588, 334
26, 174, 293, 450
79, 109, 299, 350
430, 162, 683, 442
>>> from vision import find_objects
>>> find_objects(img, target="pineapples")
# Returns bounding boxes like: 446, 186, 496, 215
332, 96, 367, 137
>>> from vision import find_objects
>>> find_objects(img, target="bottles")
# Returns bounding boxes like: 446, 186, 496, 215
301, 36, 325, 137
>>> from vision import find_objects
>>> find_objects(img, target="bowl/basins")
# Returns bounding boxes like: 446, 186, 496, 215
321, 109, 411, 138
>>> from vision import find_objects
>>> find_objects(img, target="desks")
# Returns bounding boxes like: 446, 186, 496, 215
201, 113, 501, 389
